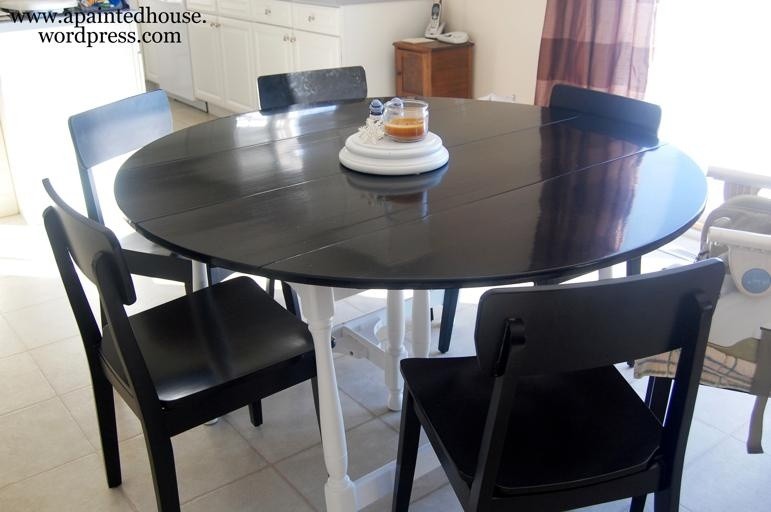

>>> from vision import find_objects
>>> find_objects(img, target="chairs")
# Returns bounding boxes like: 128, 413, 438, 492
257, 65, 369, 112
69, 89, 302, 325
391, 257, 730, 512
646, 192, 771, 456
40, 177, 340, 512
436, 82, 663, 355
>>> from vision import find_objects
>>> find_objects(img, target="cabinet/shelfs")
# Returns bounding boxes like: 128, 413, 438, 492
192, 0, 255, 120
136, 3, 191, 105
255, 0, 387, 97
394, 38, 475, 99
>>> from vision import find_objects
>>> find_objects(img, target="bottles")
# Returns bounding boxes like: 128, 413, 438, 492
369, 100, 383, 120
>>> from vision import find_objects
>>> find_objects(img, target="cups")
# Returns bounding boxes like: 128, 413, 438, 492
382, 99, 430, 144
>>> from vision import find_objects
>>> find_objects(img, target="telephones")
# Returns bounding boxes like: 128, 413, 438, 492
424, 0, 445, 38
